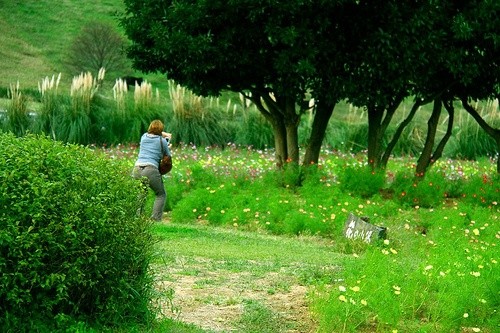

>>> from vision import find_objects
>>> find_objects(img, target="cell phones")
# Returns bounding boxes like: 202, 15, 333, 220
164, 136, 170, 146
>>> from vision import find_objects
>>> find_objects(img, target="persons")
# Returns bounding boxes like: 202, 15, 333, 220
132, 119, 173, 223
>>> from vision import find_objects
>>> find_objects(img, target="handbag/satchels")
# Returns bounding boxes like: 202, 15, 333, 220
160, 155, 172, 174
